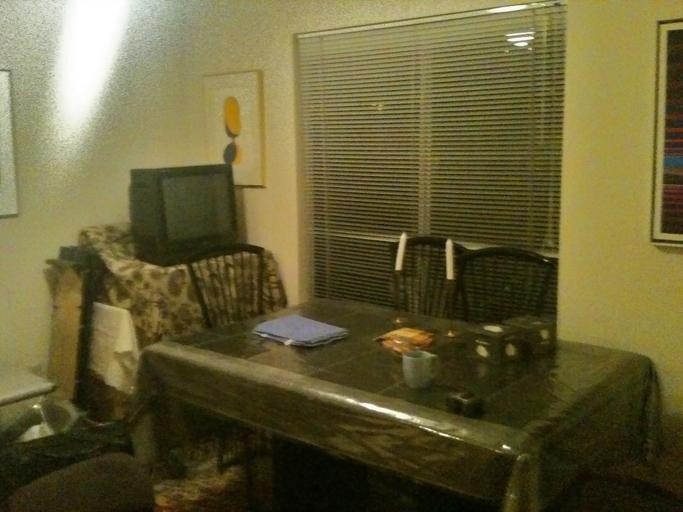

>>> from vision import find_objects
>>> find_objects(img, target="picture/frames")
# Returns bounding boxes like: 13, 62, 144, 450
0, 70, 17, 218
202, 71, 267, 188
650, 18, 682, 250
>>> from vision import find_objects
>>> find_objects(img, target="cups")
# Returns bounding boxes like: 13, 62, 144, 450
401, 349, 441, 391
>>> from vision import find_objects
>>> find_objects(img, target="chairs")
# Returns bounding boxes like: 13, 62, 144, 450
186, 243, 263, 329
388, 234, 555, 325
0, 300, 154, 512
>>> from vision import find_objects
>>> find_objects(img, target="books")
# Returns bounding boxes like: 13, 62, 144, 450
253, 311, 349, 347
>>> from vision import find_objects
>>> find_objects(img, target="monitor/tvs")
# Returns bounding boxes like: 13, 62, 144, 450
128, 163, 239, 266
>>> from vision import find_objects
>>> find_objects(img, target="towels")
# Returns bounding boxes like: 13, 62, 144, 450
89, 301, 142, 395
